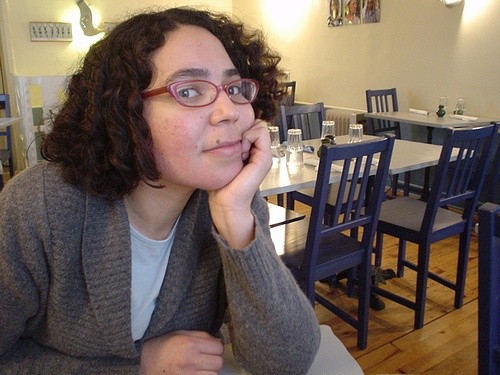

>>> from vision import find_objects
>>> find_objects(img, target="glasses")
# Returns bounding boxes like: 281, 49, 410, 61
141, 78, 261, 107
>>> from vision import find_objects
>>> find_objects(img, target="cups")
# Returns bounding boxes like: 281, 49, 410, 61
455, 98, 465, 118
320, 120, 336, 142
439, 96, 448, 112
268, 126, 280, 157
286, 129, 303, 165
348, 124, 363, 144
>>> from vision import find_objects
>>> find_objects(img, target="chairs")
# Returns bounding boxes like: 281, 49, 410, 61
264, 82, 500, 375
0, 94, 16, 177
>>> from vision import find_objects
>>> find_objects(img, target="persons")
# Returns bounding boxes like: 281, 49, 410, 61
0, 7, 320, 375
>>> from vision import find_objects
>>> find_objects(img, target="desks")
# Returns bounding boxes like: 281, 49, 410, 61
0, 117, 22, 130
258, 132, 475, 308
365, 108, 498, 198
